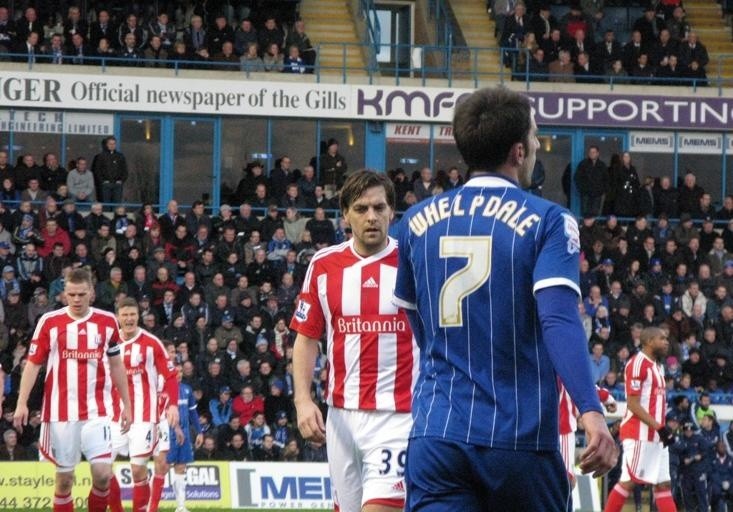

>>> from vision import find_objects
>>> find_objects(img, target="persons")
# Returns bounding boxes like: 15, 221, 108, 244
390, 85, 619, 511
485, 0, 732, 91
282, 167, 422, 511
0, 0, 318, 74
601, 459, 732, 512
108, 295, 181, 511
0, 132, 733, 461
557, 374, 620, 490
150, 338, 184, 511
148, 362, 206, 511
10, 269, 135, 511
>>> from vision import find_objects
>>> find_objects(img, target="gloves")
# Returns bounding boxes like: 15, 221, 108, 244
658, 427, 675, 447
